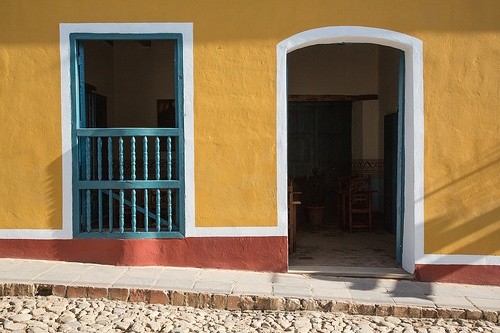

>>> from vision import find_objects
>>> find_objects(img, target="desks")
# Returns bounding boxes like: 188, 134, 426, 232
334, 186, 379, 224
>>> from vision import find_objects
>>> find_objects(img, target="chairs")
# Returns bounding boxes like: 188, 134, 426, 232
346, 173, 373, 232
